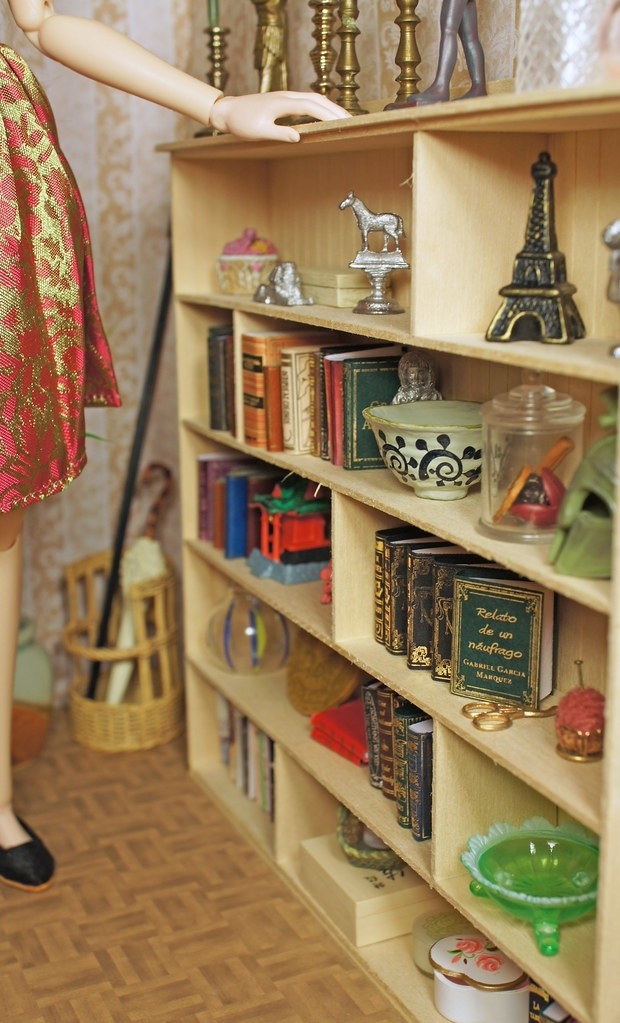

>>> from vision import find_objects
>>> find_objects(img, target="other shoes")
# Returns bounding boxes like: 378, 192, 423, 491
0, 816, 57, 892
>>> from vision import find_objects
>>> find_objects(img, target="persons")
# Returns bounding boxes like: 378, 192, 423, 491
0, 0, 355, 892
406, 0, 488, 105
391, 351, 446, 405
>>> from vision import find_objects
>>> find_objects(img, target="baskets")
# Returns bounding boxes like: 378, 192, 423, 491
336, 803, 407, 870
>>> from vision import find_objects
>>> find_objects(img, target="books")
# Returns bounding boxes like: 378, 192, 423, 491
360, 683, 434, 845
197, 449, 285, 560
525, 977, 579, 1023
371, 528, 559, 712
212, 687, 275, 825
205, 330, 404, 470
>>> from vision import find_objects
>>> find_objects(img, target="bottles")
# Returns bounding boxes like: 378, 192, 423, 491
475, 383, 586, 544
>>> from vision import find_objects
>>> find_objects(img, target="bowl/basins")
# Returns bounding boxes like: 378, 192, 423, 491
362, 399, 485, 501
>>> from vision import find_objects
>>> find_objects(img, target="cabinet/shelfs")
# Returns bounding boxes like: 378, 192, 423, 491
152, 82, 620, 1023
